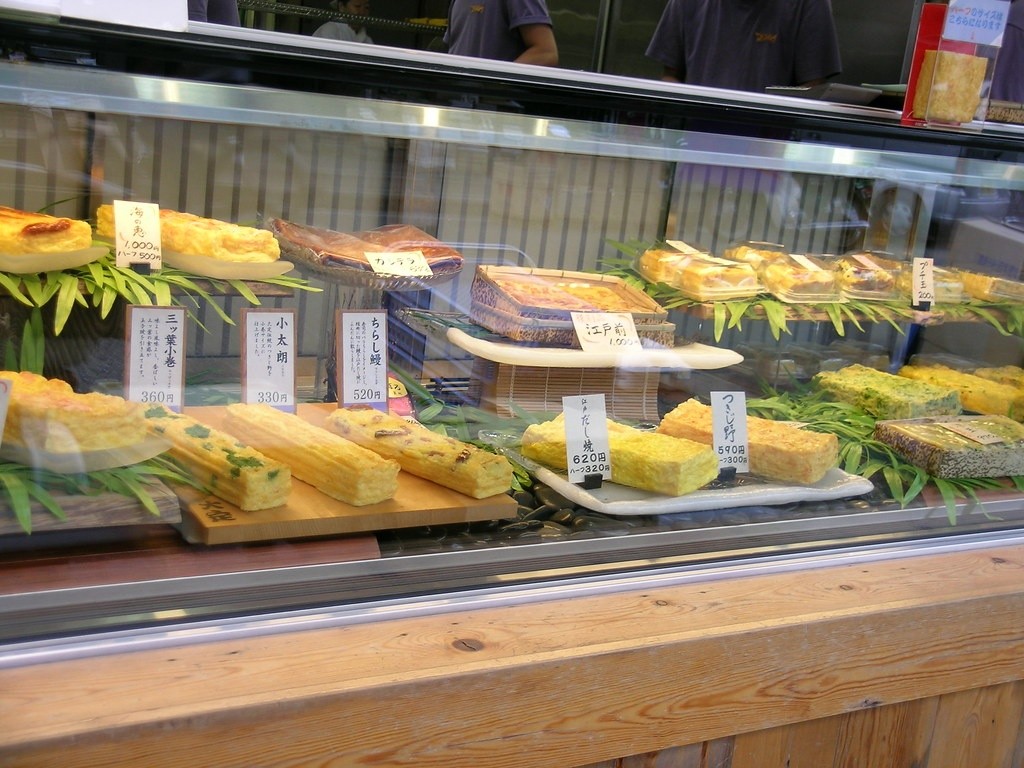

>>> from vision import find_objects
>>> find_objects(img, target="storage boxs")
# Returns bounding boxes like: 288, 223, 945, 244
921, 217, 1024, 368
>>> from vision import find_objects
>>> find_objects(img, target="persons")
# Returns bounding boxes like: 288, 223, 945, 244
643, 0, 843, 182
311, 0, 392, 100
184, 0, 247, 85
441, 0, 568, 108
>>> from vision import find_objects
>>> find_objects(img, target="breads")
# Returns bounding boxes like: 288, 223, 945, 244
913, 51, 988, 123
0, 203, 1024, 512
409, 17, 449, 28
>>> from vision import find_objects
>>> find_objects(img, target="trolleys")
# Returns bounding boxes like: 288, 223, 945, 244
378, 242, 537, 413
